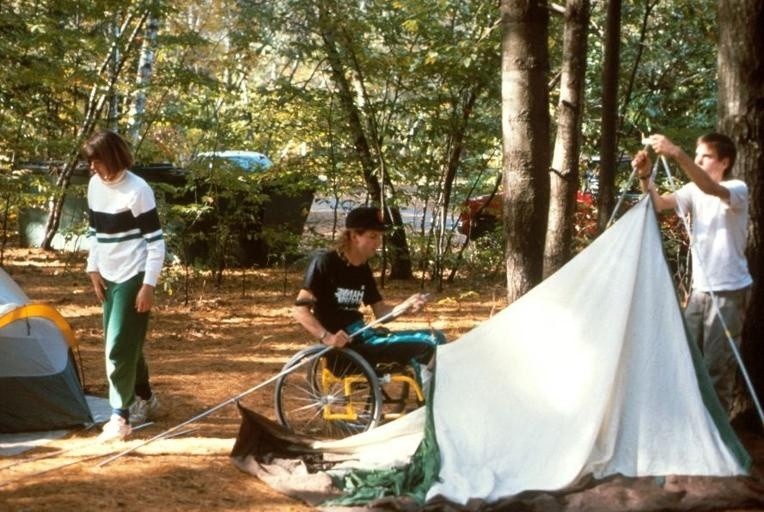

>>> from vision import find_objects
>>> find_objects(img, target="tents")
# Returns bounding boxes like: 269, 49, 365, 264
230, 192, 764, 511
1, 266, 102, 435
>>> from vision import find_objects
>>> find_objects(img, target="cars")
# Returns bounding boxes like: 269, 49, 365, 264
458, 154, 643, 249
193, 150, 274, 172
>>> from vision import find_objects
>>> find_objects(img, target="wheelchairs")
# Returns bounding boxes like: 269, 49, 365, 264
274, 344, 425, 440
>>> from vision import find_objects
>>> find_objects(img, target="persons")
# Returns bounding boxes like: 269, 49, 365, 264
80, 132, 167, 444
290, 206, 446, 404
631, 131, 753, 422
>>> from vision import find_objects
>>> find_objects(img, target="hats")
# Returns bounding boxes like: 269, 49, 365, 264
346, 207, 389, 230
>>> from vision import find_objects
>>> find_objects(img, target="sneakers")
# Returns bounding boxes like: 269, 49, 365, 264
96, 412, 132, 443
129, 392, 160, 424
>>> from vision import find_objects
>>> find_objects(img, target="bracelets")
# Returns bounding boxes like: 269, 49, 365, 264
319, 331, 331, 341
638, 173, 651, 180
